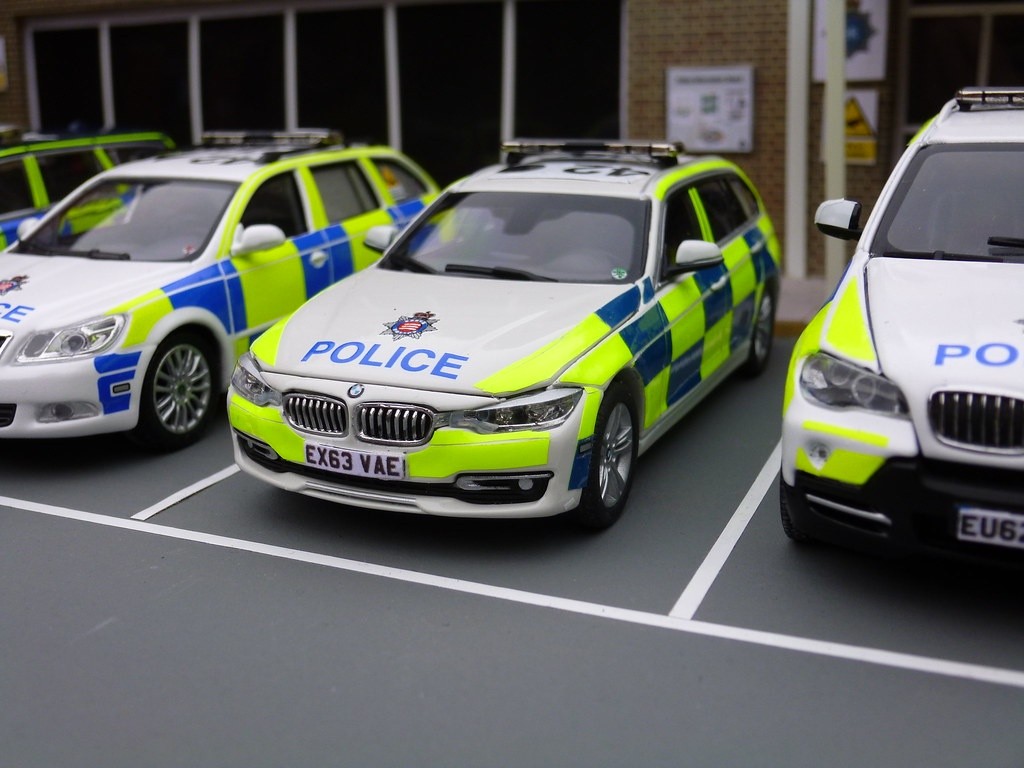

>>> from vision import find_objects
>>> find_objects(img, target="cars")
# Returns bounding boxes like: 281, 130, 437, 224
0, 126, 440, 456
228, 139, 784, 538
0, 126, 180, 252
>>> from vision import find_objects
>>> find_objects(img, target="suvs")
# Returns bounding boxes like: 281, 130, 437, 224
780, 84, 1024, 589
